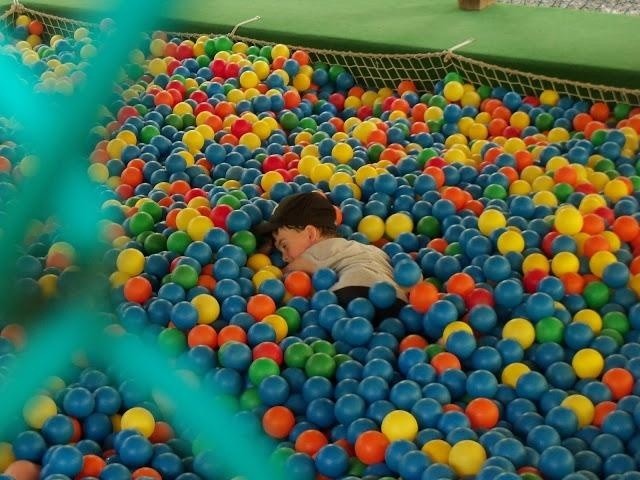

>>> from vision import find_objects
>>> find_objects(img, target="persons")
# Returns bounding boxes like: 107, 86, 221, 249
265, 191, 423, 344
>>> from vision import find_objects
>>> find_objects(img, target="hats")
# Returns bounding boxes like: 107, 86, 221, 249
257, 192, 335, 232
